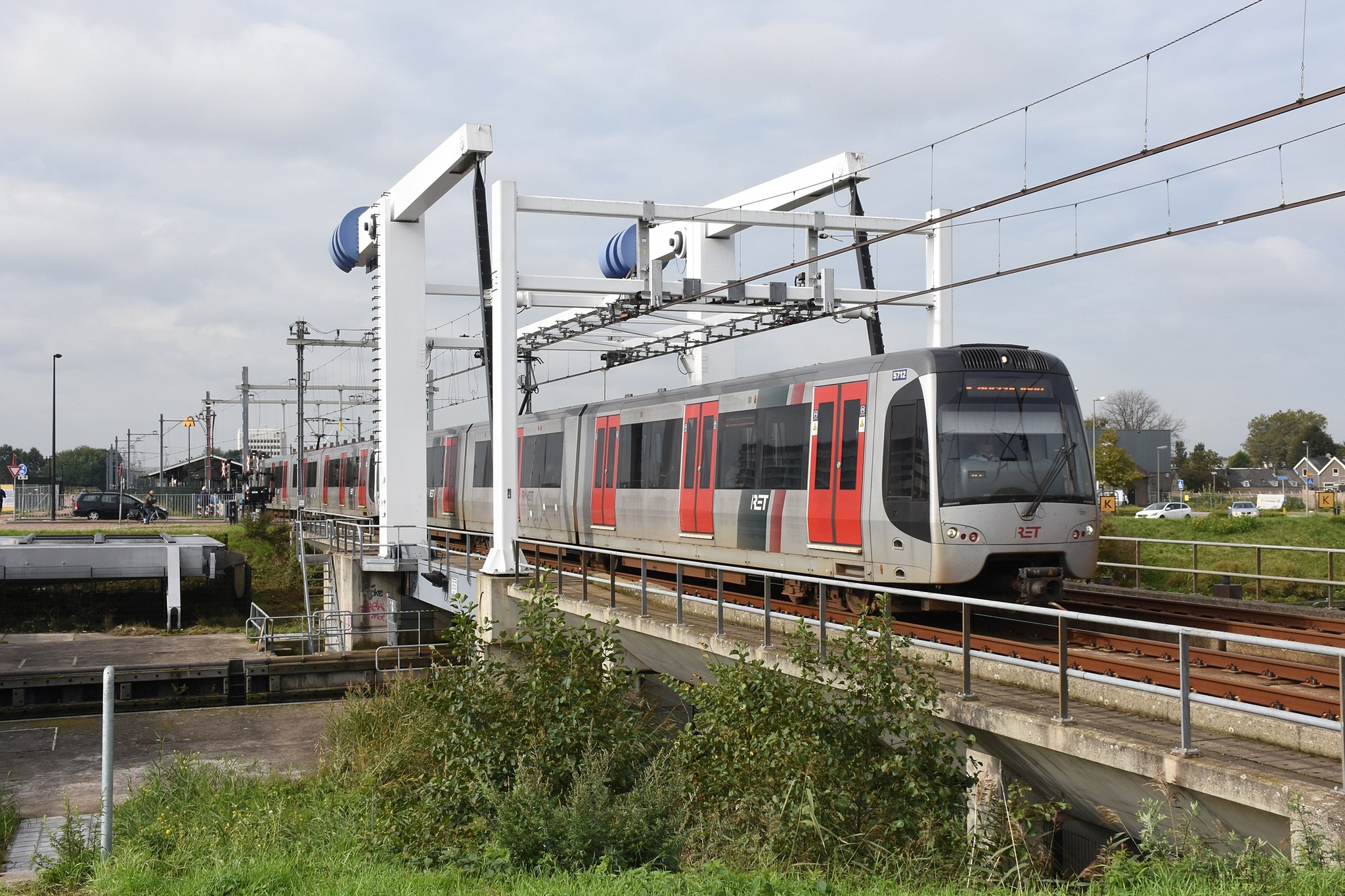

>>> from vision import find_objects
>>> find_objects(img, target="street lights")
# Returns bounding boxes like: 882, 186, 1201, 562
1157, 446, 1167, 503
1303, 441, 1308, 516
51, 353, 63, 520
1210, 471, 1217, 493
1093, 396, 1106, 477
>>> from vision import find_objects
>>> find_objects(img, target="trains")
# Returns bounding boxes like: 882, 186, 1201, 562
260, 341, 1102, 622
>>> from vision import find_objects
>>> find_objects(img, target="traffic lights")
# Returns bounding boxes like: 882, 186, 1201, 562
421, 571, 446, 587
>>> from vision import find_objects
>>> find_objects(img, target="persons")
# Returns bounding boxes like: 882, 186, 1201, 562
143, 490, 158, 524
199, 486, 208, 516
966, 436, 1000, 461
0, 485, 6, 512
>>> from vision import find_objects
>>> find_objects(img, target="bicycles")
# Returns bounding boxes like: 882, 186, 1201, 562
127, 501, 167, 526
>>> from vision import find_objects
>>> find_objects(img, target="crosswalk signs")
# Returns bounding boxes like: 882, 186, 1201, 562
1178, 480, 1183, 491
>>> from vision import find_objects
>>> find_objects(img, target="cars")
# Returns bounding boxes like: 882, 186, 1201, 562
1228, 501, 1261, 519
1135, 502, 1192, 521
1097, 490, 1129, 508
71, 491, 169, 521
